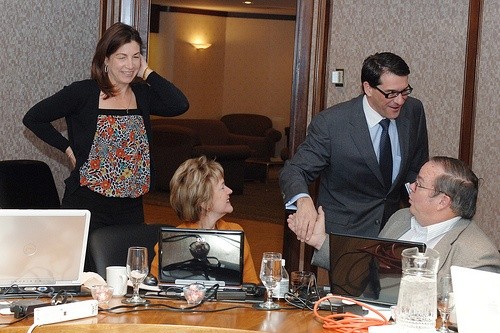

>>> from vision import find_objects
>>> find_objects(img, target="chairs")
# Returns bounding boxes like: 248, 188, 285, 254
0, 160, 61, 210
90, 224, 168, 277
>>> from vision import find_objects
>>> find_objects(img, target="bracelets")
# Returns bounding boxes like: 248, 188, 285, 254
67, 153, 73, 160
143, 67, 149, 78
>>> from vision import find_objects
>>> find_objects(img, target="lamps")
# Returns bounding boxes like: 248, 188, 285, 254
192, 43, 212, 51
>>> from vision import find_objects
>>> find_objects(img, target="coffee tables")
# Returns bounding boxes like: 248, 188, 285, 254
244, 157, 284, 192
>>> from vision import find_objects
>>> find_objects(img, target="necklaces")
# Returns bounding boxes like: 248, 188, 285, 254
115, 90, 131, 113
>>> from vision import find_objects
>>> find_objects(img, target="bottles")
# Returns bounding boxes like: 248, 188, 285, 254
271, 258, 290, 302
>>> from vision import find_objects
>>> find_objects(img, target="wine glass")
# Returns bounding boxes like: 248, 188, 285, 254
126, 247, 149, 303
435, 274, 458, 333
259, 251, 283, 309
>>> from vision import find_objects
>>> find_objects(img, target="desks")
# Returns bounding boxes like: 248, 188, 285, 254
0, 290, 453, 333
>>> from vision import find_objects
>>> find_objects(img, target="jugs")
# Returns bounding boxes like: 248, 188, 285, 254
394, 247, 440, 333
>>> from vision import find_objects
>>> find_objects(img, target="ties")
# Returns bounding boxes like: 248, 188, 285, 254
378, 118, 393, 195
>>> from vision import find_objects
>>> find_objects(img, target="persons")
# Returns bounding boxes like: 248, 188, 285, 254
150, 155, 259, 285
278, 52, 430, 238
286, 156, 500, 296
22, 22, 190, 229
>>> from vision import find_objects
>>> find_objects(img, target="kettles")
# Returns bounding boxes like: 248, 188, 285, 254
106, 265, 127, 297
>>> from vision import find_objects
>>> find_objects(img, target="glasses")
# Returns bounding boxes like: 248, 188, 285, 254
368, 82, 413, 99
410, 177, 453, 202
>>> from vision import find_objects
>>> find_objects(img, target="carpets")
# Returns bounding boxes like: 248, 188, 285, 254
142, 178, 286, 225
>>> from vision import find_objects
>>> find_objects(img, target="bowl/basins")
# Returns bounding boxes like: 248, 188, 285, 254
91, 284, 114, 306
182, 284, 207, 305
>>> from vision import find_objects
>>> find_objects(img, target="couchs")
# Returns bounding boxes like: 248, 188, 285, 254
150, 113, 282, 196
